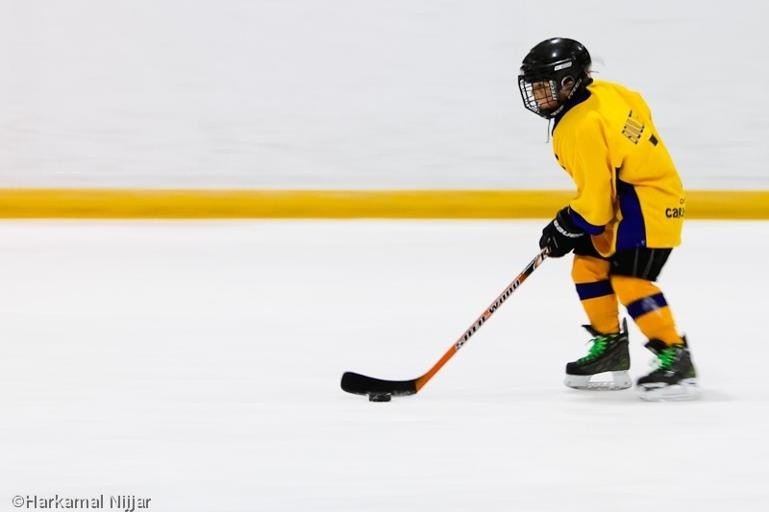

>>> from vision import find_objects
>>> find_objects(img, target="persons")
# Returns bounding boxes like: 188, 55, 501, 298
517, 33, 700, 392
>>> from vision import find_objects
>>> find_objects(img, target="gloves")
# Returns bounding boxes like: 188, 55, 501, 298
540, 207, 586, 257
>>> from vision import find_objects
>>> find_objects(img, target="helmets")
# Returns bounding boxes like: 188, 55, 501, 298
521, 37, 591, 83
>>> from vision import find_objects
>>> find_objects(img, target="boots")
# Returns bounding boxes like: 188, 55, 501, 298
566, 317, 630, 375
637, 335, 695, 388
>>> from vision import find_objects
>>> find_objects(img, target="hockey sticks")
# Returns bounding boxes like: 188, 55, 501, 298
340, 245, 550, 396
